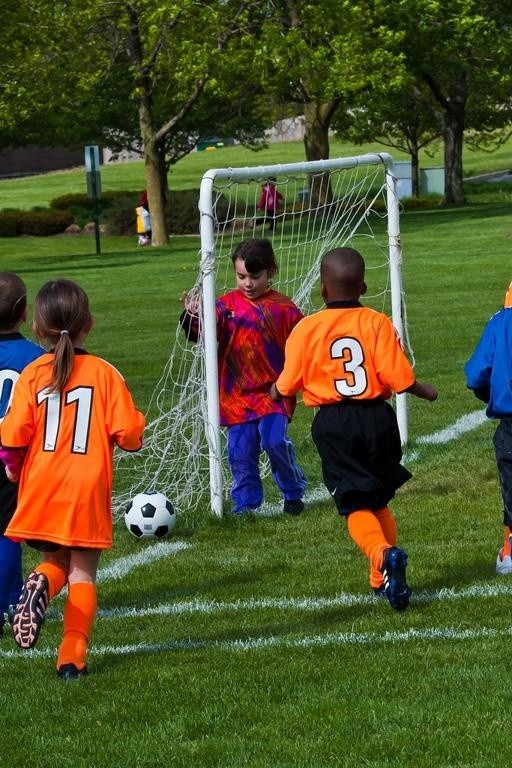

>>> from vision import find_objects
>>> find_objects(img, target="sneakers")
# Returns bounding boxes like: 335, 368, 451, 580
379, 547, 414, 613
373, 582, 386, 599
11, 570, 50, 650
495, 545, 511, 575
58, 662, 88, 681
283, 498, 304, 518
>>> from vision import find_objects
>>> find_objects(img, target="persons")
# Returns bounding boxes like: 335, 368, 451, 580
134, 189, 151, 246
460, 280, 511, 580
0, 277, 149, 682
176, 236, 309, 520
0, 270, 49, 636
250, 175, 285, 232
268, 244, 438, 609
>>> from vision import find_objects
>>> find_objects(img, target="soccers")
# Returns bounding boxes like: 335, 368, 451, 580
125, 492, 176, 542
